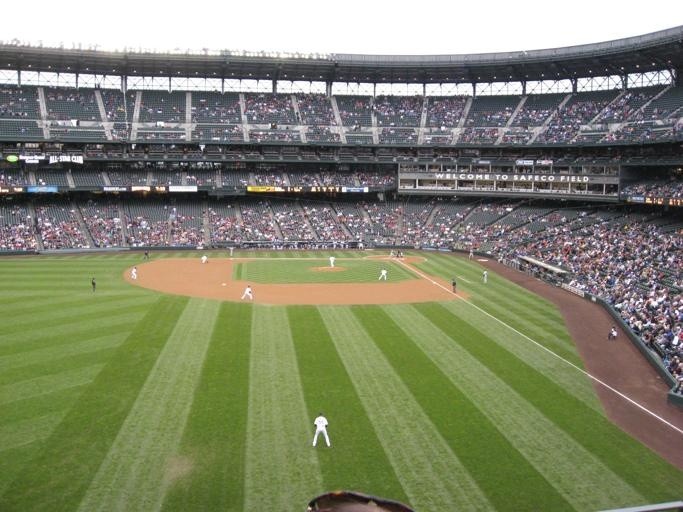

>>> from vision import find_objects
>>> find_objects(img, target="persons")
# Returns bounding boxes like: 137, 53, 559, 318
90, 249, 150, 292
603, 302, 681, 402
379, 250, 403, 280
201, 254, 208, 263
330, 256, 335, 267
483, 268, 487, 284
469, 249, 474, 260
241, 285, 254, 300
0, 79, 682, 251
311, 412, 330, 447
452, 278, 457, 292
498, 251, 682, 304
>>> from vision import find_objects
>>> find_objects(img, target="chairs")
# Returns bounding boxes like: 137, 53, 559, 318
0, 83, 683, 303
